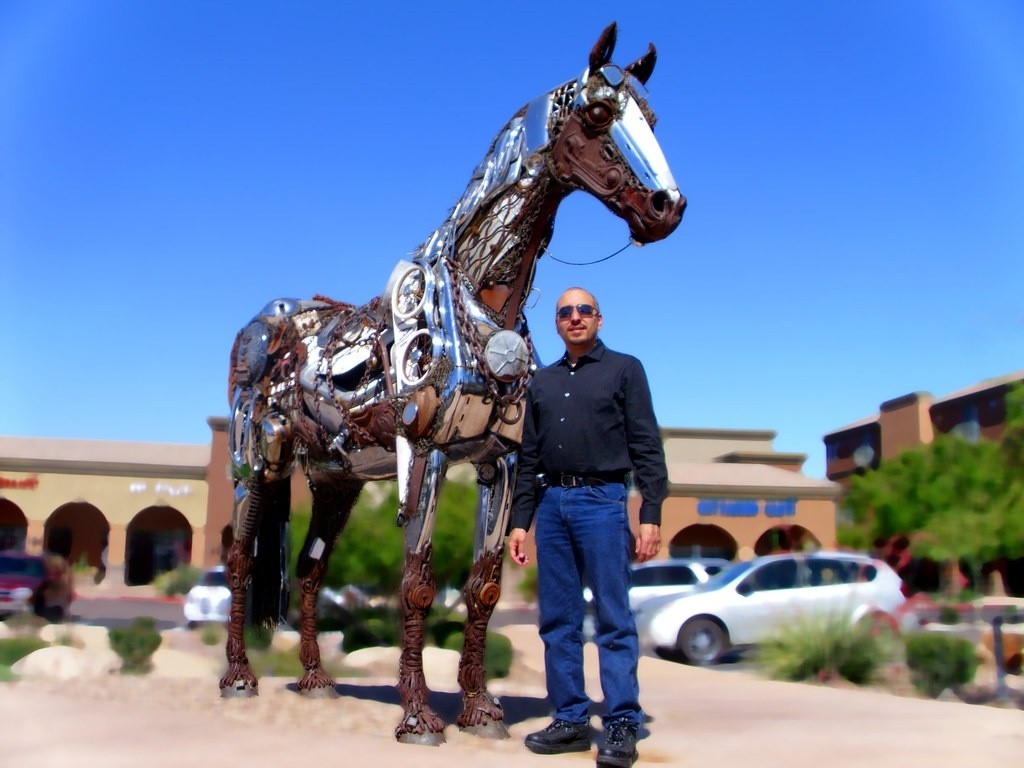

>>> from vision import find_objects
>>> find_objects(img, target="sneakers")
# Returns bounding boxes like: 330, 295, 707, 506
524, 720, 591, 754
596, 723, 639, 768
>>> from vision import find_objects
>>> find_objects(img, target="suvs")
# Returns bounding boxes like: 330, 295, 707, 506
585, 558, 751, 615
637, 553, 921, 664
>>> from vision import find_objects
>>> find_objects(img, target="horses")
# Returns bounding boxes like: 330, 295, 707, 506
219, 22, 686, 747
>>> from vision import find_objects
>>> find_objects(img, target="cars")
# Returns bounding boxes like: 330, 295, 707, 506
0, 552, 74, 624
908, 592, 1023, 676
185, 566, 370, 630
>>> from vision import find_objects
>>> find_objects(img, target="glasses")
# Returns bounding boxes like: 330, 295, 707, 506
556, 304, 600, 318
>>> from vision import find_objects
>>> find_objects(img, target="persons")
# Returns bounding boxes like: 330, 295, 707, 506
504, 288, 670, 768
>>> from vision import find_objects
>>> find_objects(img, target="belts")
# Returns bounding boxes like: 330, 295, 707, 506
546, 473, 627, 488
